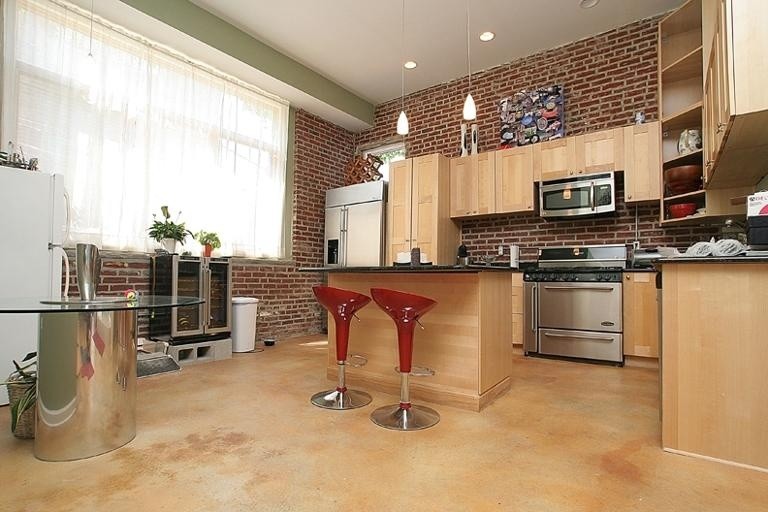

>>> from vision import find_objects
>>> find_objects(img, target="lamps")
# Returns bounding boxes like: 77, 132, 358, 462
463, 0, 476, 121
397, 0, 409, 135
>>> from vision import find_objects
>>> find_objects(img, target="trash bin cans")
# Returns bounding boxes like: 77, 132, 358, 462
232, 297, 259, 352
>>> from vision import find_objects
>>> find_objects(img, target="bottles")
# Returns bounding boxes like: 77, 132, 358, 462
76, 242, 102, 303
411, 248, 421, 266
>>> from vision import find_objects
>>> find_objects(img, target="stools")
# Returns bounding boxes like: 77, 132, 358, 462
370, 287, 441, 431
311, 285, 372, 410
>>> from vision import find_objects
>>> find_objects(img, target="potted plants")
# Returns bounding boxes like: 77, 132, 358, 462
145, 206, 194, 254
193, 229, 221, 257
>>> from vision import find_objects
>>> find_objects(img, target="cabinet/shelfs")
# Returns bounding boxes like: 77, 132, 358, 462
496, 144, 534, 214
511, 272, 524, 355
449, 151, 495, 219
623, 272, 659, 368
657, 0, 767, 228
384, 153, 460, 269
534, 127, 624, 182
624, 121, 661, 203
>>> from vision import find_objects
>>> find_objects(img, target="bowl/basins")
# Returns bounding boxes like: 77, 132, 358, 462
263, 339, 276, 346
663, 164, 703, 196
668, 203, 697, 218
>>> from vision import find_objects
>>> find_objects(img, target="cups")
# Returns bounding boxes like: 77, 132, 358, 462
635, 111, 646, 125
457, 256, 469, 267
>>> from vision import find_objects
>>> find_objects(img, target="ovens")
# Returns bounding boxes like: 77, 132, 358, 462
522, 281, 624, 367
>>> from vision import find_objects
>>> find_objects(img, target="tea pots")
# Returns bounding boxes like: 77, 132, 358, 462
677, 129, 702, 157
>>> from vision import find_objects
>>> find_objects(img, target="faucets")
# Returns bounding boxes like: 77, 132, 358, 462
724, 217, 746, 234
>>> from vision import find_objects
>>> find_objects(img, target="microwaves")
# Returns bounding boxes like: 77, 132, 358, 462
539, 171, 616, 222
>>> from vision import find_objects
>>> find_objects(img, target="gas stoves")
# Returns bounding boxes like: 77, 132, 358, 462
525, 266, 625, 281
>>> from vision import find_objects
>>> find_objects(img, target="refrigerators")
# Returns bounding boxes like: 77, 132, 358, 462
322, 180, 388, 335
0, 164, 71, 407
